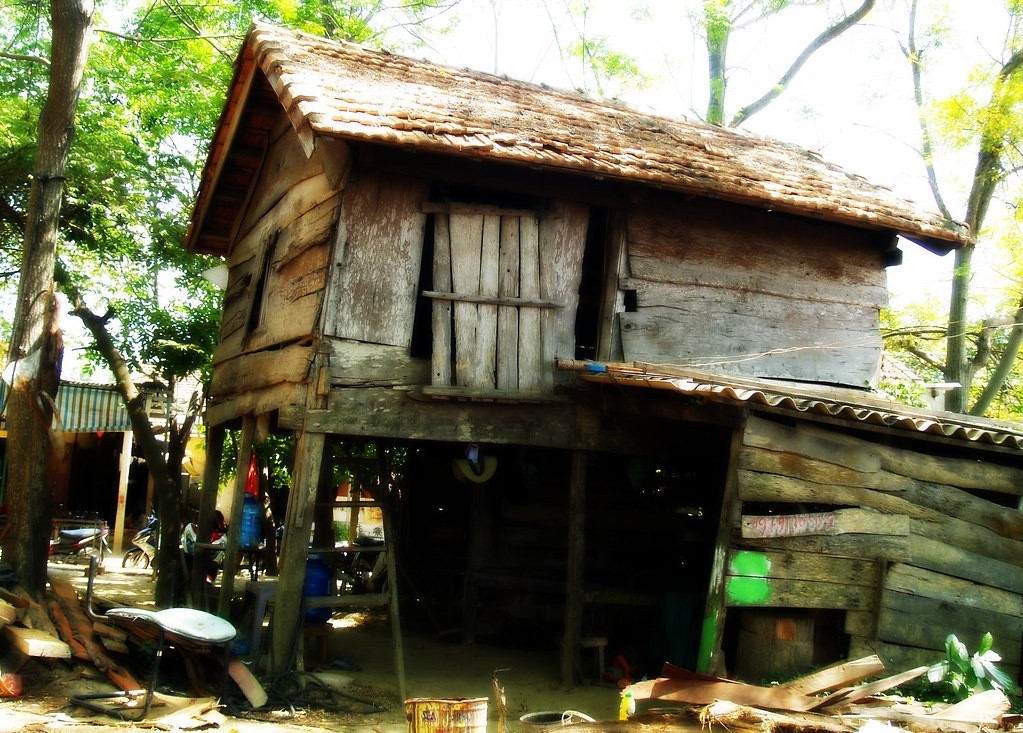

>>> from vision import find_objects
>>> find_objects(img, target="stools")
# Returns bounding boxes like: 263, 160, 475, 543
243, 581, 277, 663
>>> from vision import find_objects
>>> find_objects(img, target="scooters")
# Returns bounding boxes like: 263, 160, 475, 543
48, 527, 112, 566
182, 509, 229, 585
121, 508, 159, 568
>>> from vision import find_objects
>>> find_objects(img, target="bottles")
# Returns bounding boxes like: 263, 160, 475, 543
240, 493, 262, 548
304, 554, 332, 621
